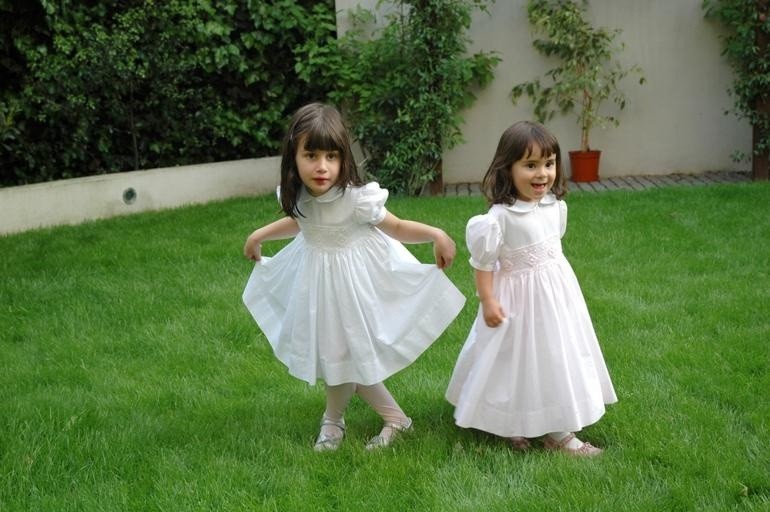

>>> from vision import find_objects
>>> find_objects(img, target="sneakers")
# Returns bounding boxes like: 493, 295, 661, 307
507, 437, 534, 453
313, 414, 347, 455
544, 432, 604, 459
365, 416, 414, 453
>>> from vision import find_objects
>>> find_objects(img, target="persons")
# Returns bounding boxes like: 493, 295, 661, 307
239, 100, 469, 452
444, 121, 619, 457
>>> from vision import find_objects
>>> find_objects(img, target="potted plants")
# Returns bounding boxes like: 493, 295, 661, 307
505, 0, 648, 183
